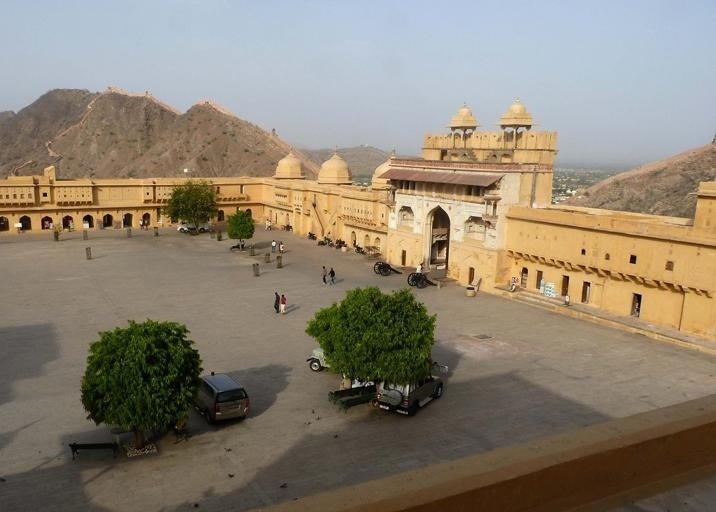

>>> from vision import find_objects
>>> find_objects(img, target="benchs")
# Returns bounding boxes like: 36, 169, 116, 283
336, 394, 378, 415
233, 246, 244, 248
328, 385, 375, 405
69, 443, 118, 460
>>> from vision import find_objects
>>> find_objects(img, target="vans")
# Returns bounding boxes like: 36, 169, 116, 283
377, 370, 444, 416
191, 370, 249, 423
176, 217, 209, 233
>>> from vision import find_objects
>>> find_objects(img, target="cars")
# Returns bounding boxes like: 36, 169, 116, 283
306, 347, 332, 371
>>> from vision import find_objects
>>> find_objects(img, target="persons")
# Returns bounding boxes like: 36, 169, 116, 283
264, 218, 269, 232
138, 218, 143, 230
68, 219, 75, 232
278, 241, 284, 254
280, 294, 287, 314
268, 220, 272, 231
320, 266, 327, 285
328, 267, 336, 287
271, 239, 276, 252
274, 291, 280, 314
